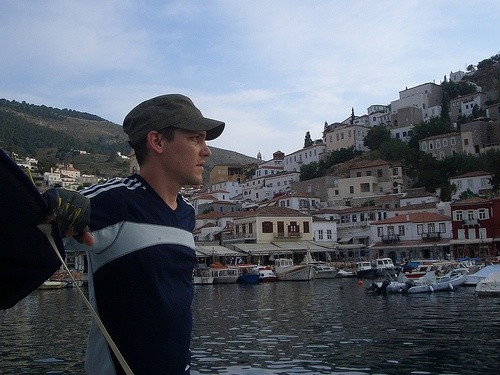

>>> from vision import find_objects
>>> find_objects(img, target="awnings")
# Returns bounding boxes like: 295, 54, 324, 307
338, 238, 352, 243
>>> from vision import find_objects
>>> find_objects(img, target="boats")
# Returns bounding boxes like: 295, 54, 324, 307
36, 261, 84, 290
192, 255, 500, 299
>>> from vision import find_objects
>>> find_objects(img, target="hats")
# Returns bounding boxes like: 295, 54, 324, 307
123, 94, 225, 147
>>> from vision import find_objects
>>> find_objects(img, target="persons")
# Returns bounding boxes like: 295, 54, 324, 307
42, 93, 225, 375
